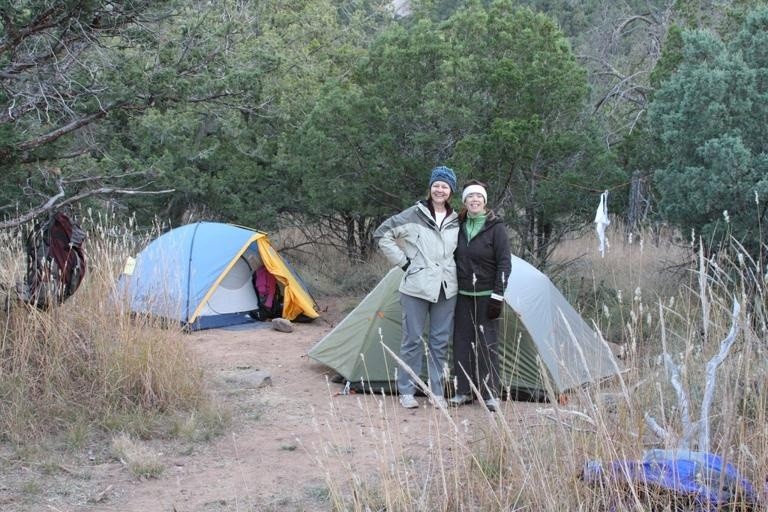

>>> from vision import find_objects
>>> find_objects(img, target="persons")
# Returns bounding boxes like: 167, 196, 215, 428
447, 179, 512, 412
373, 166, 462, 409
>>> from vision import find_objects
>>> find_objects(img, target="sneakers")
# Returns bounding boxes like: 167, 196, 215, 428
450, 394, 474, 407
428, 394, 448, 410
398, 392, 420, 409
485, 397, 501, 411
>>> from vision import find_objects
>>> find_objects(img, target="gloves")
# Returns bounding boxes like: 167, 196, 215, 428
487, 293, 505, 319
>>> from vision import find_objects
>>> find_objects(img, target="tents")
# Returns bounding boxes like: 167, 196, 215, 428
109, 219, 320, 332
301, 253, 639, 405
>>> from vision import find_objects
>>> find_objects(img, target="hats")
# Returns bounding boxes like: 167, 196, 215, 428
429, 166, 457, 194
463, 179, 489, 206
